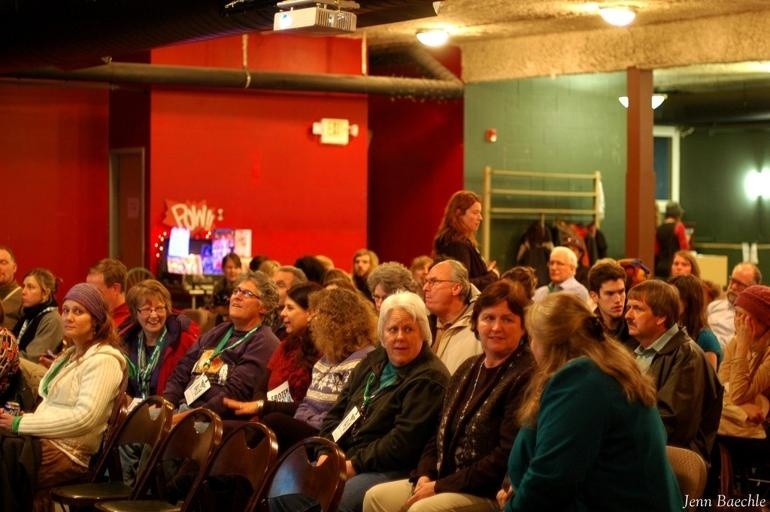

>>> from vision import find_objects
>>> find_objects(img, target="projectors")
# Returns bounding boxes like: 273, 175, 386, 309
274, 8, 357, 35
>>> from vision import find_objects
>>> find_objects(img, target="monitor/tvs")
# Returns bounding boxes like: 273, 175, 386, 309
653, 125, 680, 214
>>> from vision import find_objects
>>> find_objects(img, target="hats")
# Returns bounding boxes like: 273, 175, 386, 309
733, 285, 769, 326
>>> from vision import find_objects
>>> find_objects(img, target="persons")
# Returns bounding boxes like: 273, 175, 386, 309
1, 247, 27, 331
654, 205, 659, 266
1, 267, 66, 364
1, 284, 128, 511
434, 191, 500, 287
654, 203, 692, 279
85, 242, 769, 512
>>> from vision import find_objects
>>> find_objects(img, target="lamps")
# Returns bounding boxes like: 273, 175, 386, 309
594, 4, 641, 28
414, 23, 453, 51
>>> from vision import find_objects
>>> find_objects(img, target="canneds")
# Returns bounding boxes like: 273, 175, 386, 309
4, 401, 20, 416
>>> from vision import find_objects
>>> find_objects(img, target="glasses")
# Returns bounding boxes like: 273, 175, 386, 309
232, 288, 261, 301
423, 278, 455, 286
136, 306, 165, 315
